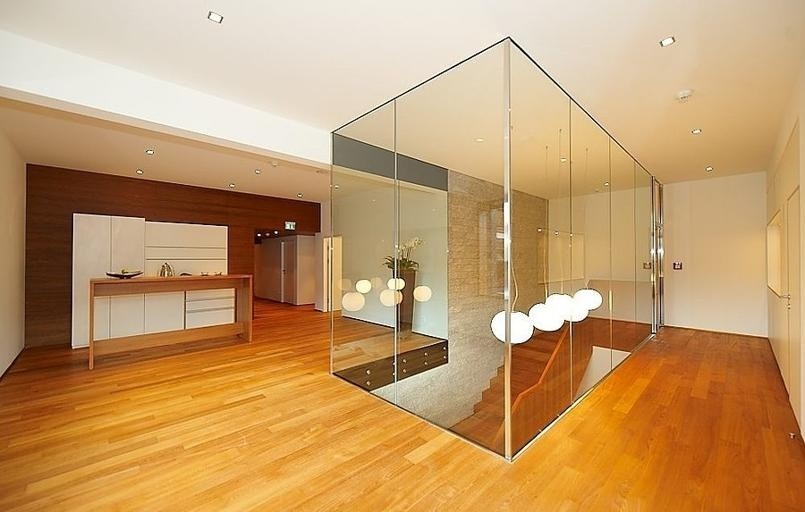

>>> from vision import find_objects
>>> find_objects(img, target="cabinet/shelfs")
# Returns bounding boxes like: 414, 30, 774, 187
69, 212, 236, 350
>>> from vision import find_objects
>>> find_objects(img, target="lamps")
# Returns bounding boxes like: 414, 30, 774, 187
490, 128, 603, 344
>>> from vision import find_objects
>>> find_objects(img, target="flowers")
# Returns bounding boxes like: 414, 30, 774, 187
381, 237, 424, 270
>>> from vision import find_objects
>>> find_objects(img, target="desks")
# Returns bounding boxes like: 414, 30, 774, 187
88, 274, 253, 369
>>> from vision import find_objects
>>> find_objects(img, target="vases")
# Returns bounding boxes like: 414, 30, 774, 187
392, 270, 416, 339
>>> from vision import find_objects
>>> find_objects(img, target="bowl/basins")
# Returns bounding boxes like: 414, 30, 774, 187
200, 271, 222, 276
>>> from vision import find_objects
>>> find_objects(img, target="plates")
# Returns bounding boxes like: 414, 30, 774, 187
104, 271, 144, 279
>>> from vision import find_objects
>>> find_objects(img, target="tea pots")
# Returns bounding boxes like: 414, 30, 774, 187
160, 262, 173, 277
